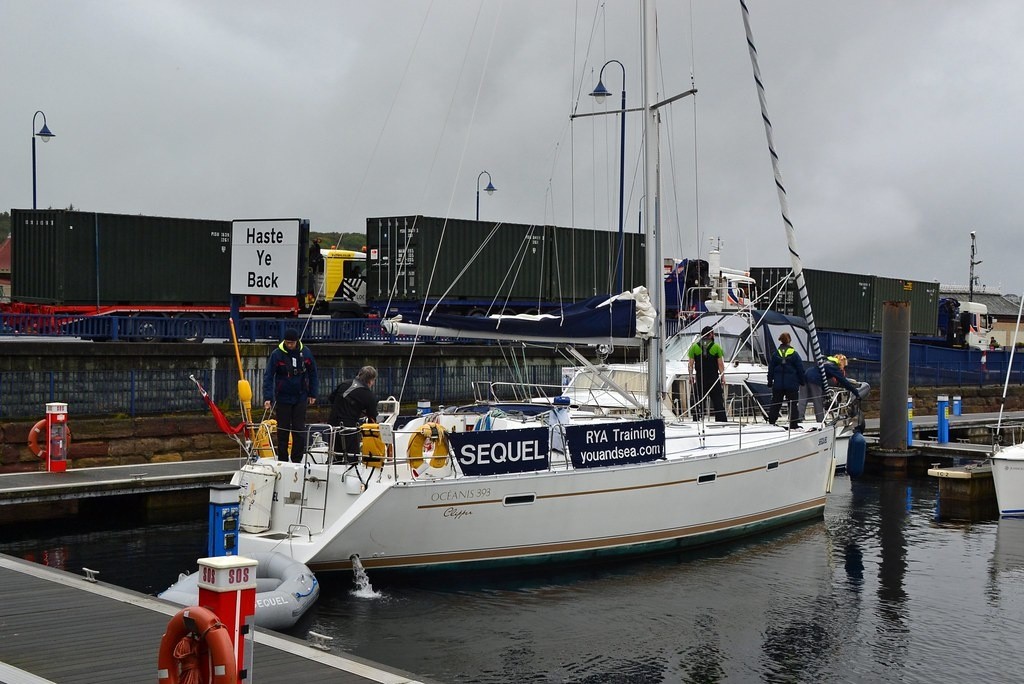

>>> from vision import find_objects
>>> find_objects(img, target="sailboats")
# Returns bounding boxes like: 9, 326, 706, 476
987, 293, 1024, 518
228, 0, 857, 571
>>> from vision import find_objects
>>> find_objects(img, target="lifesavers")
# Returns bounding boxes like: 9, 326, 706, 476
29, 418, 71, 457
408, 422, 449, 469
259, 419, 294, 458
158, 607, 237, 684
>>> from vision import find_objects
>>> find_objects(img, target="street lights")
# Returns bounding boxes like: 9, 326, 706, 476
476, 171, 497, 221
32, 111, 56, 209
589, 60, 626, 294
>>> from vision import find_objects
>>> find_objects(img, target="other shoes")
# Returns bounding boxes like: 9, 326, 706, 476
798, 426, 803, 429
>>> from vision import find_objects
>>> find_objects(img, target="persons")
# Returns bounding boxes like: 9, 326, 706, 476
687, 326, 727, 422
767, 333, 808, 429
989, 337, 999, 348
798, 354, 863, 422
262, 328, 319, 463
325, 366, 380, 465
309, 237, 323, 298
349, 265, 366, 299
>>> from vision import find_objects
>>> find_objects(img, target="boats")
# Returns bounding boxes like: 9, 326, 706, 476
157, 549, 320, 630
561, 227, 826, 422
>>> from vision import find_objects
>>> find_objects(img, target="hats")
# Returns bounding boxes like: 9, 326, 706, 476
284, 328, 299, 341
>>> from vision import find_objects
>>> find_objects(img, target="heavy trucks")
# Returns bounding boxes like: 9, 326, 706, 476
749, 267, 996, 351
10, 208, 367, 321
367, 215, 709, 335
